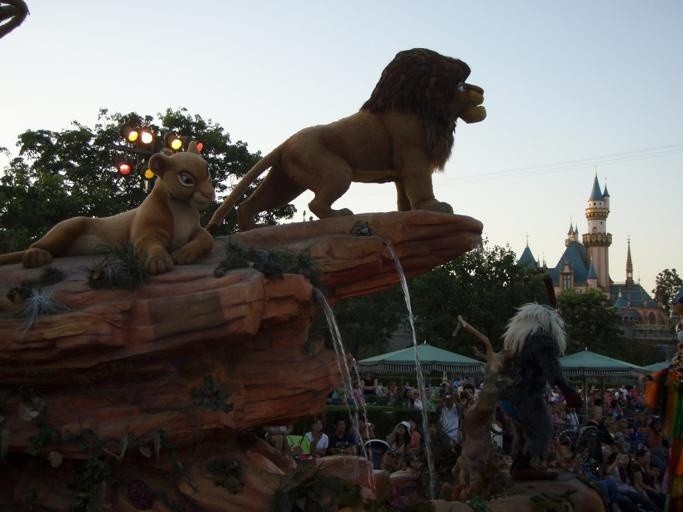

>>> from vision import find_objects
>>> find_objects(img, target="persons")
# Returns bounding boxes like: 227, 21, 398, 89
264, 369, 671, 512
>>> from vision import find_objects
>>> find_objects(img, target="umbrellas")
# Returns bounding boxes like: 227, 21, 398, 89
557, 346, 647, 414
645, 362, 671, 377
356, 340, 485, 389
